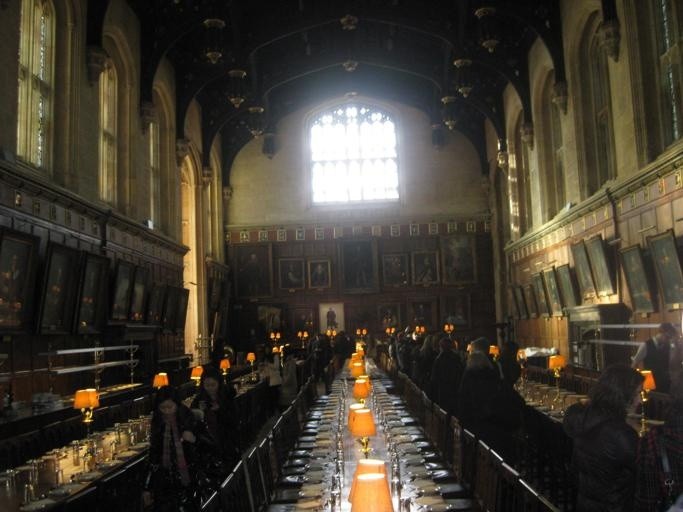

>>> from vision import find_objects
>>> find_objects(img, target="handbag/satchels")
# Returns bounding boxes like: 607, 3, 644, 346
139, 469, 158, 512
657, 480, 682, 511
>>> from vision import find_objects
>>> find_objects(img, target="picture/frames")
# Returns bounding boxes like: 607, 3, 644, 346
226, 219, 479, 344
500, 223, 682, 321
0, 220, 189, 345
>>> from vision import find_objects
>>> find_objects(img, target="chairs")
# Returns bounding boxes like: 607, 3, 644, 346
0, 312, 683, 511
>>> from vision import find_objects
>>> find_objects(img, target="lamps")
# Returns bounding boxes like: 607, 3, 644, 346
338, 5, 360, 104
475, 8, 500, 52
262, 122, 279, 159
441, 94, 457, 130
225, 60, 246, 110
454, 55, 473, 98
431, 122, 445, 153
204, 16, 225, 64
247, 98, 264, 140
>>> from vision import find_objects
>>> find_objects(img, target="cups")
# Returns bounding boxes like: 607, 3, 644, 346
516, 378, 588, 422
0, 415, 150, 509
297, 378, 454, 512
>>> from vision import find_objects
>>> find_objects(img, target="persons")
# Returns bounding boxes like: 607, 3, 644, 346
633, 349, 682, 511
324, 306, 340, 333
559, 364, 647, 511
388, 331, 525, 466
630, 321, 678, 393
140, 386, 220, 511
238, 237, 473, 297
252, 329, 348, 419
187, 368, 242, 475
